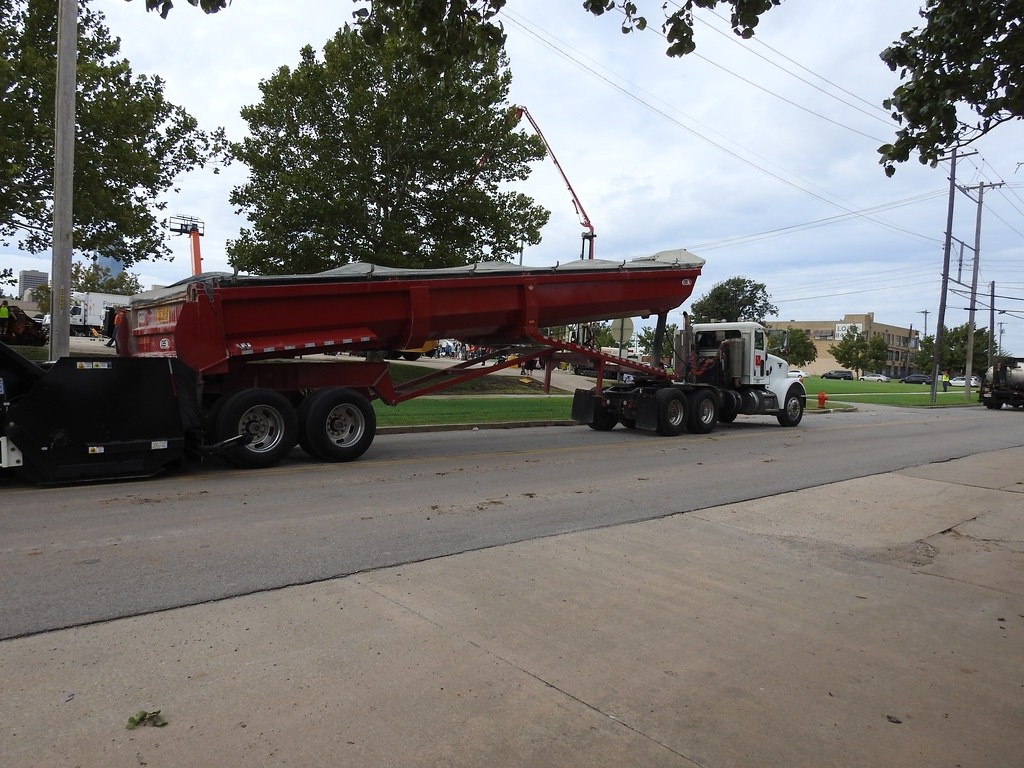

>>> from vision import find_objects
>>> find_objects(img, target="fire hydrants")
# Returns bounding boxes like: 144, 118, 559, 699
818, 389, 828, 409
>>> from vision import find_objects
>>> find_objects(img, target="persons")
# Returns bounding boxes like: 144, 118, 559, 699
0, 300, 11, 334
106, 305, 124, 347
451, 342, 475, 361
526, 358, 535, 376
480, 347, 486, 366
941, 369, 949, 392
521, 362, 527, 375
445, 343, 450, 355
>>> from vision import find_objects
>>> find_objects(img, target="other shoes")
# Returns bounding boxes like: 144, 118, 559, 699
104, 343, 111, 347
112, 345, 116, 348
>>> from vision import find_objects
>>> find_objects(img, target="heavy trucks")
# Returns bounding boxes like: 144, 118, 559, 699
0, 245, 809, 489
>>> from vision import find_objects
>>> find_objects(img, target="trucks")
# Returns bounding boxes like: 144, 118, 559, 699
43, 289, 132, 327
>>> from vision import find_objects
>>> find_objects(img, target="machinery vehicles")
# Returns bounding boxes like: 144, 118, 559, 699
981, 356, 1024, 410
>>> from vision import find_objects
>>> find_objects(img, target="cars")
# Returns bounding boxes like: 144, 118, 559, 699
787, 369, 811, 379
947, 377, 981, 387
898, 375, 932, 385
820, 369, 853, 381
858, 373, 891, 383
